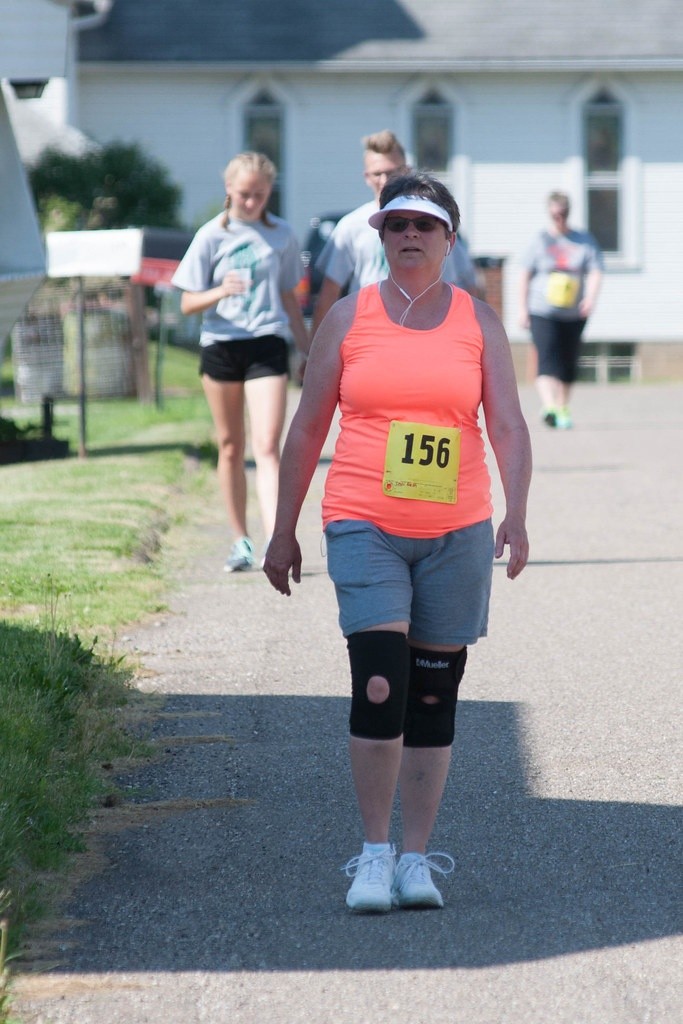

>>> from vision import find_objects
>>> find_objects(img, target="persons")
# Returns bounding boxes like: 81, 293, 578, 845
264, 169, 532, 912
514, 192, 604, 431
170, 150, 310, 573
310, 131, 476, 333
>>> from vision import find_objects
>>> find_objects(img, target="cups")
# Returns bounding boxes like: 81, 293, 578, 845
231, 268, 251, 297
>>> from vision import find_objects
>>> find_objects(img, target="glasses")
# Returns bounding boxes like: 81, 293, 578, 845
550, 206, 569, 219
382, 213, 438, 234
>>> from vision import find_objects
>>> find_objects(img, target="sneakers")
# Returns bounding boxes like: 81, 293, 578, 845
392, 852, 456, 908
542, 404, 572, 430
345, 846, 396, 913
226, 537, 253, 571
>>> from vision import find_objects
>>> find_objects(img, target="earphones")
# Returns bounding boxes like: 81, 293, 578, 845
446, 240, 451, 255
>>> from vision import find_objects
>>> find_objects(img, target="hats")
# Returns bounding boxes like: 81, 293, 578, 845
368, 195, 453, 235
